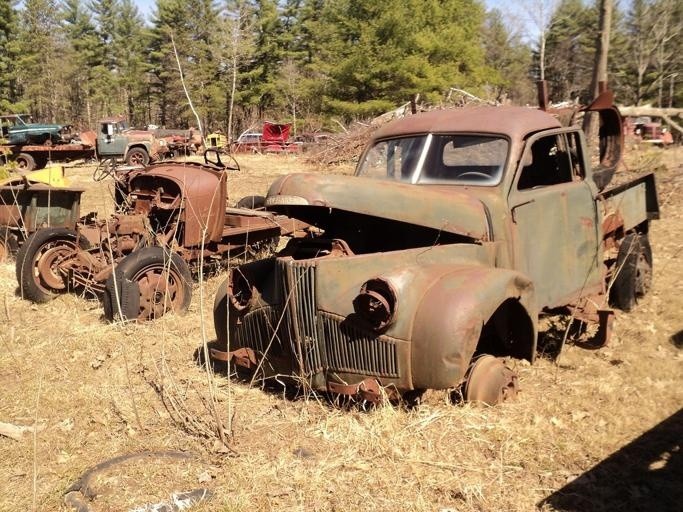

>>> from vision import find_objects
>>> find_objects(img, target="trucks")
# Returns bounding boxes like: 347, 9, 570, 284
0, 112, 191, 171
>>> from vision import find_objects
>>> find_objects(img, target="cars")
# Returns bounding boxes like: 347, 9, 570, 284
622, 117, 672, 146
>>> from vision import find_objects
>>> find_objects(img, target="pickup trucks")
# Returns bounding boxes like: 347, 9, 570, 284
193, 80, 660, 407
